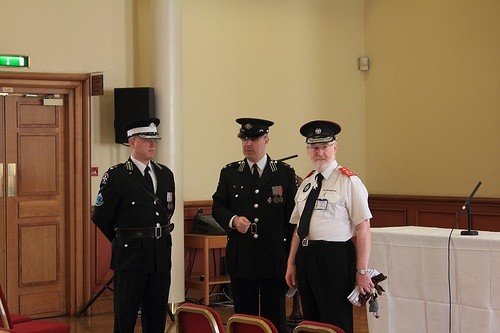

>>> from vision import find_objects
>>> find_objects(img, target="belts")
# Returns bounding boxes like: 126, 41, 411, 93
250, 222, 289, 235
302, 238, 350, 246
117, 223, 175, 239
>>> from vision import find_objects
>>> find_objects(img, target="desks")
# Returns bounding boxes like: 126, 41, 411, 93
365, 225, 500, 333
184, 232, 232, 308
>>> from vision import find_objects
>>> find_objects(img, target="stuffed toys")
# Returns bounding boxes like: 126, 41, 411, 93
368, 288, 380, 319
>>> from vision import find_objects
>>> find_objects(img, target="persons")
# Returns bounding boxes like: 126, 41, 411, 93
285, 120, 374, 333
211, 118, 298, 333
91, 117, 176, 333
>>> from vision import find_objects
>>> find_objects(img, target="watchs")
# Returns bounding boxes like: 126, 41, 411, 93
356, 268, 369, 275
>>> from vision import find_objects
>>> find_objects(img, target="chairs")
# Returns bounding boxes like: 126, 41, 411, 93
226, 314, 278, 333
0, 285, 71, 333
292, 321, 344, 333
175, 303, 226, 333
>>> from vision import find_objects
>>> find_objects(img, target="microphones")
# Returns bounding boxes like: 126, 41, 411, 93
461, 182, 482, 212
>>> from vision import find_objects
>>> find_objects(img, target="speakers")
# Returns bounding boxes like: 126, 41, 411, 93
114, 87, 155, 144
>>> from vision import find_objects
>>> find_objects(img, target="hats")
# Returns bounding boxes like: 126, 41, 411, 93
236, 118, 275, 139
122, 118, 162, 139
299, 119, 341, 144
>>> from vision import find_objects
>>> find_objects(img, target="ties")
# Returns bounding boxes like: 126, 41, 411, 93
144, 166, 154, 194
252, 163, 259, 186
297, 173, 324, 243
